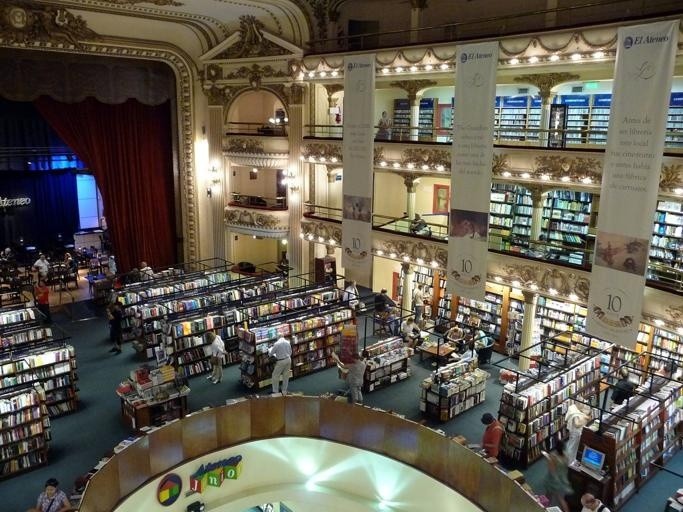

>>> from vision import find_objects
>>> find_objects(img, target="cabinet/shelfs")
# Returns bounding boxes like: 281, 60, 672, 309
95, 256, 368, 433
389, 96, 683, 144
488, 185, 683, 294
398, 262, 681, 511
359, 333, 413, 394
0, 291, 82, 483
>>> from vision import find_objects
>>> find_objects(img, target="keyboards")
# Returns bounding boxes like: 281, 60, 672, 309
579, 465, 605, 482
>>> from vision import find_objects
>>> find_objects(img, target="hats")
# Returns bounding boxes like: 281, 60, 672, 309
481, 412, 494, 424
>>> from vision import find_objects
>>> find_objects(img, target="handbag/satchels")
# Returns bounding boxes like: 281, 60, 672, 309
210, 357, 219, 366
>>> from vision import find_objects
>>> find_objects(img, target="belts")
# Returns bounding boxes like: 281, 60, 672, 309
276, 356, 288, 361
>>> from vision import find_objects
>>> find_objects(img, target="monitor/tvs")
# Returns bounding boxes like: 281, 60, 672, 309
581, 444, 606, 471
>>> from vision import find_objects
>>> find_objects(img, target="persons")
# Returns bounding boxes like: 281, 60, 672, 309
60, 252, 75, 274
35, 479, 72, 512
108, 256, 117, 275
339, 353, 366, 403
345, 280, 359, 325
76, 246, 105, 266
0, 246, 19, 280
31, 254, 50, 280
373, 111, 392, 142
139, 261, 154, 281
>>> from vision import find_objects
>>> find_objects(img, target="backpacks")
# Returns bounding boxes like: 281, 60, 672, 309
491, 420, 508, 448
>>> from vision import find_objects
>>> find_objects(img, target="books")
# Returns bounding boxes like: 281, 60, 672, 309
67, 434, 145, 512
362, 261, 683, 512
391, 103, 683, 149
488, 180, 600, 273
92, 266, 352, 433
1, 279, 80, 478
645, 197, 682, 292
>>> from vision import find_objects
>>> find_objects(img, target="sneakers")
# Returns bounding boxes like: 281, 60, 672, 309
205, 375, 220, 384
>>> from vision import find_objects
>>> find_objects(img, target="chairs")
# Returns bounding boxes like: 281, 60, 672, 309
1, 238, 113, 291
